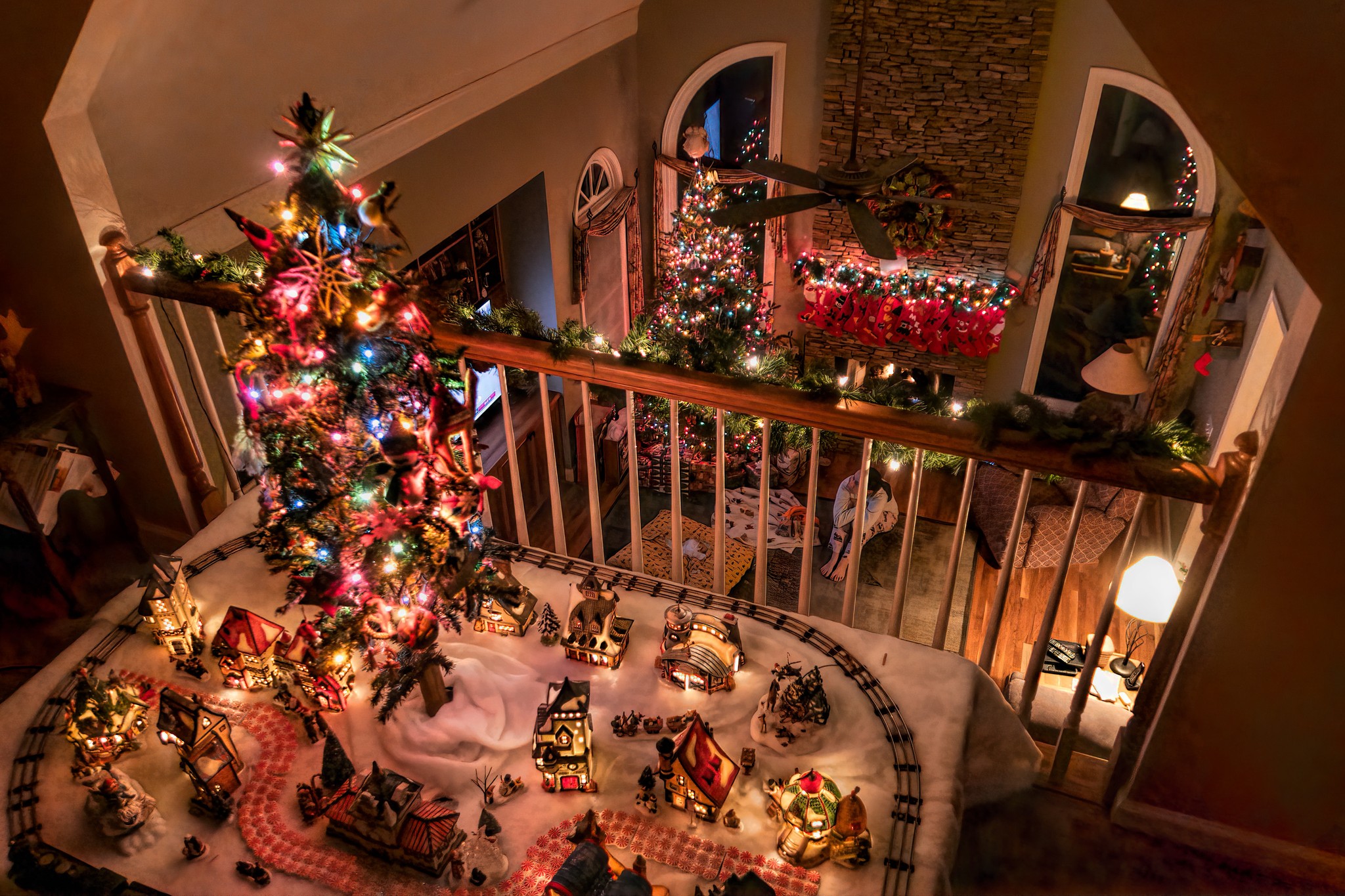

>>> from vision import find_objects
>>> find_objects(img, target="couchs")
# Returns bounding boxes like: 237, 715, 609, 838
973, 435, 1143, 571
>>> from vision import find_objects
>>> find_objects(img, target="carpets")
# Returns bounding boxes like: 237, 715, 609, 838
581, 473, 994, 668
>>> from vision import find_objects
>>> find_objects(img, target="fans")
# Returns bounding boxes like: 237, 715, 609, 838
706, 0, 1018, 260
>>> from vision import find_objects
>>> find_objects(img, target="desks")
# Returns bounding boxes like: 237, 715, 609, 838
1, 366, 155, 621
0, 490, 1048, 896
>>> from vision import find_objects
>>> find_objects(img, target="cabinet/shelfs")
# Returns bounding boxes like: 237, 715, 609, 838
473, 389, 569, 544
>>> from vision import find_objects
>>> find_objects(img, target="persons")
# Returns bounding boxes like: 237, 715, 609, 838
821, 466, 898, 582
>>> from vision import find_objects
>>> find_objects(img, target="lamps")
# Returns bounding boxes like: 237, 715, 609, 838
1082, 342, 1153, 426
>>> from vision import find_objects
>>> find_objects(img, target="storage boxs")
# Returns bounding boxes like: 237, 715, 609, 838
639, 432, 811, 498
